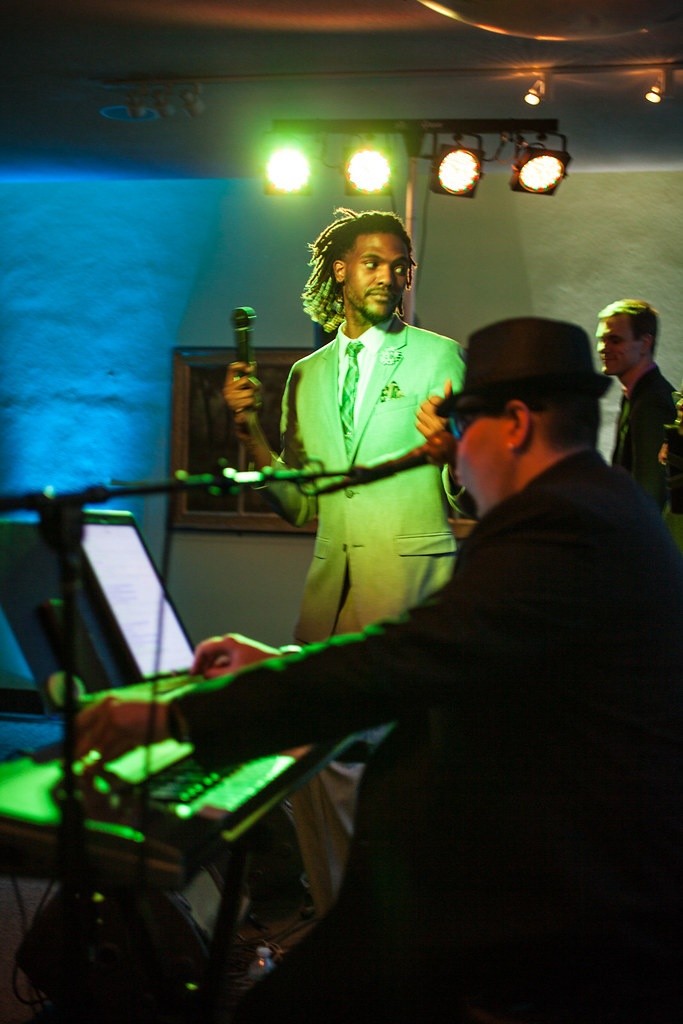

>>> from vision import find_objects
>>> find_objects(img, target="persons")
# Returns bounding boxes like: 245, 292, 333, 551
591, 298, 683, 509
220, 207, 470, 923
76, 317, 682, 1024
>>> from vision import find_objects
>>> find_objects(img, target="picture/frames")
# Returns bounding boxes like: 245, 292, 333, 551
171, 343, 478, 540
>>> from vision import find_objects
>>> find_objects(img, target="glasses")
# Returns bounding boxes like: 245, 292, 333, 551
442, 416, 479, 442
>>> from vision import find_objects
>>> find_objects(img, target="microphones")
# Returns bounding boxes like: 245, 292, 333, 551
230, 305, 256, 433
315, 430, 458, 496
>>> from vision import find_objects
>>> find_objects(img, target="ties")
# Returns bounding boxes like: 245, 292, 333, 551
340, 341, 364, 461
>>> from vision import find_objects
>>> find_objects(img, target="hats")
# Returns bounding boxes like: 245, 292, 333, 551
434, 316, 613, 418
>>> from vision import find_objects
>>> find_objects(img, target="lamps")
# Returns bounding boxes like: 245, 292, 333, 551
508, 141, 571, 198
523, 72, 547, 106
343, 147, 392, 196
646, 70, 671, 105
427, 141, 487, 196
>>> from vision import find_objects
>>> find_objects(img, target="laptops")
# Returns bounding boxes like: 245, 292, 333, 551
67, 509, 203, 682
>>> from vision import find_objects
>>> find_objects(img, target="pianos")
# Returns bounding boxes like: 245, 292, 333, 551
0, 729, 365, 940
